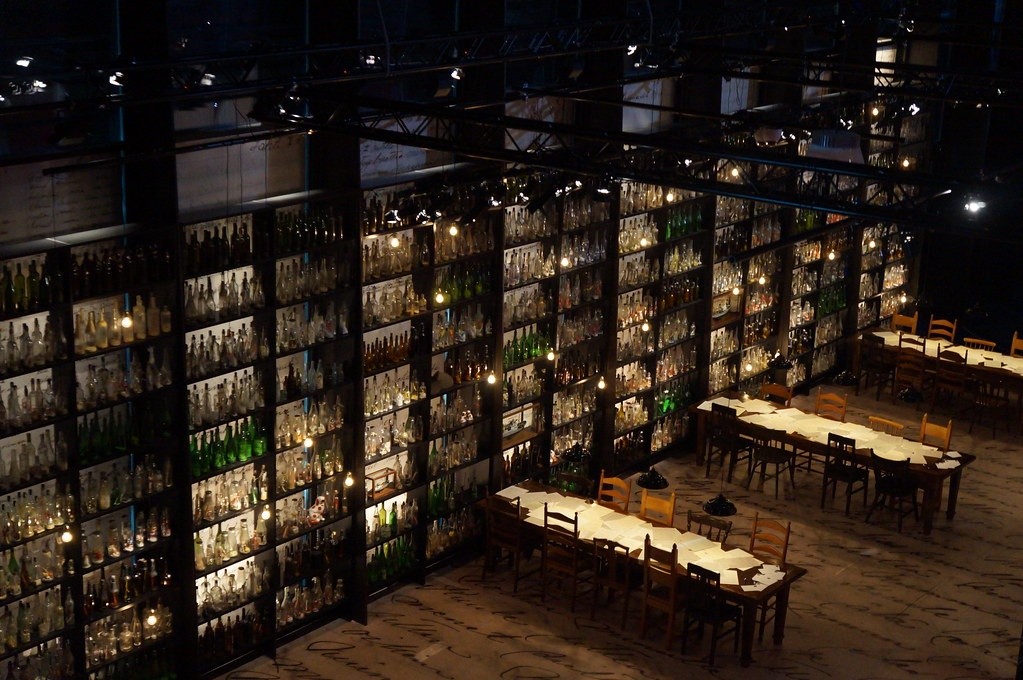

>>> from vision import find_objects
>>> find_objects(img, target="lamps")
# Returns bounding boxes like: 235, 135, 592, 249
831, 223, 860, 386
561, 444, 587, 462
765, 202, 791, 370
896, 232, 925, 402
635, 459, 669, 490
904, 20, 914, 32
703, 454, 739, 517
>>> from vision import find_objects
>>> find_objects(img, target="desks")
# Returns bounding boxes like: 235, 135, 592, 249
480, 480, 807, 668
689, 388, 980, 532
848, 326, 1023, 433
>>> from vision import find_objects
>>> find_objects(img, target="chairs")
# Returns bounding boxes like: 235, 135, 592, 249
739, 513, 791, 643
637, 534, 689, 649
480, 493, 547, 593
596, 469, 631, 515
556, 467, 595, 498
751, 374, 794, 453
679, 563, 743, 666
852, 312, 1023, 439
862, 448, 919, 534
791, 386, 848, 488
687, 509, 732, 543
819, 433, 869, 515
909, 413, 953, 512
590, 535, 643, 632
539, 502, 602, 609
747, 426, 794, 499
854, 416, 904, 488
706, 402, 755, 481
641, 488, 675, 527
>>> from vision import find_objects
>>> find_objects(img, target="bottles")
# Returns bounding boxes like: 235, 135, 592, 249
505, 169, 609, 486
186, 204, 349, 668
786, 111, 929, 388
614, 168, 701, 467
709, 152, 779, 395
362, 181, 494, 585
0, 241, 171, 680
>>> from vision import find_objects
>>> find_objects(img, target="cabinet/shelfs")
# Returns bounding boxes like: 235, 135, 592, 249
0, 93, 936, 679
